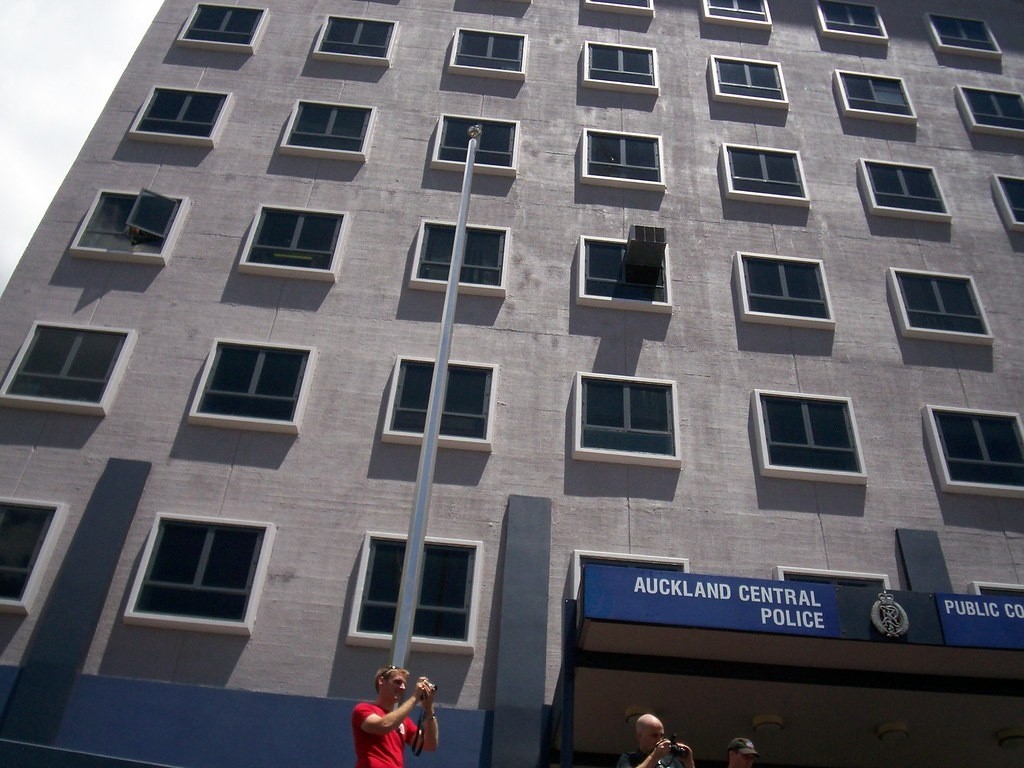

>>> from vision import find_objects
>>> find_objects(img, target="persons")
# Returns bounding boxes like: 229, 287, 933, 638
620, 714, 695, 768
351, 665, 439, 768
727, 738, 758, 768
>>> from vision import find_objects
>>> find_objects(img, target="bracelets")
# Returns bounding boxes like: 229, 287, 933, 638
425, 712, 436, 720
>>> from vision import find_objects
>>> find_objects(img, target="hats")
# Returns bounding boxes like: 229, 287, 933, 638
726, 737, 759, 757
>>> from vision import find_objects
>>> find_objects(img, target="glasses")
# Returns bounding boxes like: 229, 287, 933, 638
380, 665, 400, 676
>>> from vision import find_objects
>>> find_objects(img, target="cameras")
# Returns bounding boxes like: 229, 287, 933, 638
423, 680, 438, 692
668, 735, 688, 758
